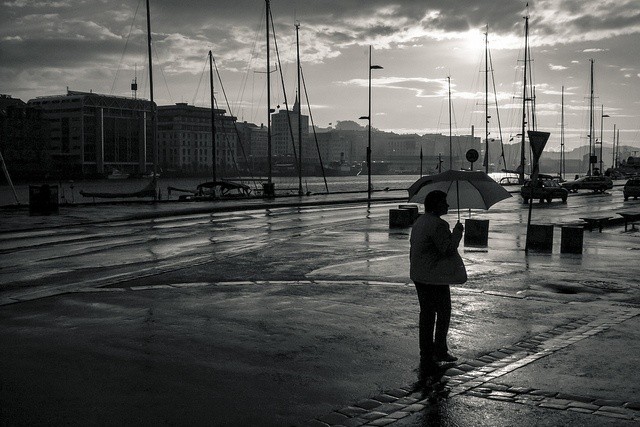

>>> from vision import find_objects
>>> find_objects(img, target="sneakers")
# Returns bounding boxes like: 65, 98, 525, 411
434, 351, 459, 364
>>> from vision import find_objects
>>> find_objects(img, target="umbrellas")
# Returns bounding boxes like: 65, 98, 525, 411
406, 168, 514, 222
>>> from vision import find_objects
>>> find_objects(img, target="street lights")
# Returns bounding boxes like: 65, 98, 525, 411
596, 104, 610, 175
517, 99, 531, 185
358, 45, 383, 208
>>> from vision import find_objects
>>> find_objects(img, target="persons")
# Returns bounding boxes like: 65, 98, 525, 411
409, 189, 467, 362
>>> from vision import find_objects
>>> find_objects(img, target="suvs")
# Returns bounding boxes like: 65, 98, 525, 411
562, 176, 613, 192
623, 179, 640, 200
521, 180, 568, 203
